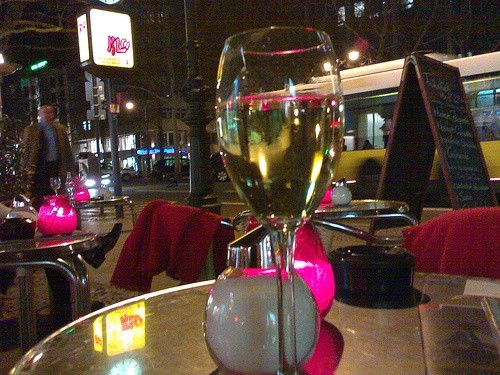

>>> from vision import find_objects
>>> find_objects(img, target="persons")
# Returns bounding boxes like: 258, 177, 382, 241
0, 203, 122, 325
20, 105, 80, 208
342, 135, 389, 150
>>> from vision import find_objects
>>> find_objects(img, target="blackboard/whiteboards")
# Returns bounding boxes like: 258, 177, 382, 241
375, 52, 498, 208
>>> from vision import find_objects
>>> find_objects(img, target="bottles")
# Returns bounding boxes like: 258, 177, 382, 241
65, 171, 75, 200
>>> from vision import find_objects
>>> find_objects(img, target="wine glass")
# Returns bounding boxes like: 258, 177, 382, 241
217, 25, 345, 375
50, 177, 61, 195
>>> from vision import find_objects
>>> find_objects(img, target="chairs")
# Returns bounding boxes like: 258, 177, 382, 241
109, 200, 235, 298
401, 207, 500, 281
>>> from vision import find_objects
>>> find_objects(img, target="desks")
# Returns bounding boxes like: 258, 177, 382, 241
0, 233, 101, 354
71, 196, 137, 230
9, 272, 500, 375
313, 199, 418, 253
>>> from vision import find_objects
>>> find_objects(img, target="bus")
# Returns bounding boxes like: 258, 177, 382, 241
256, 51, 500, 206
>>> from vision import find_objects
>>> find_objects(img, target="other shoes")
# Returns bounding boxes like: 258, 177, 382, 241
81, 222, 122, 269
54, 301, 105, 328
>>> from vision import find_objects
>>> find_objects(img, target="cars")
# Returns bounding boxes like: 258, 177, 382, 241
210, 152, 230, 182
100, 163, 137, 183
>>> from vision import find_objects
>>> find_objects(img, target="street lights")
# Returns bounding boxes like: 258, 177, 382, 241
125, 99, 151, 177
